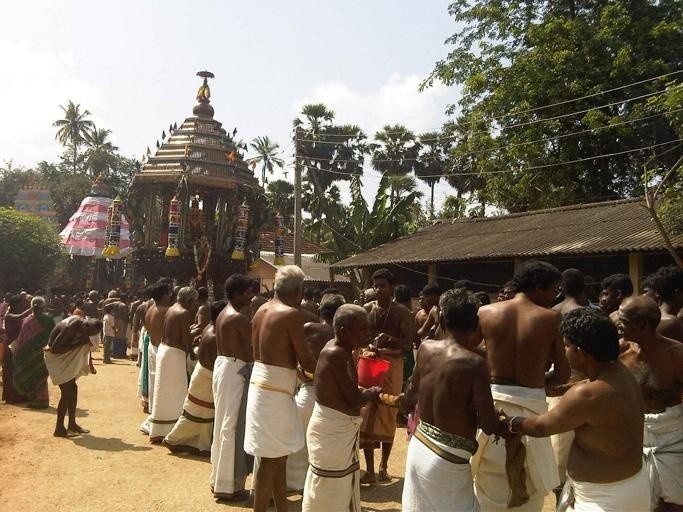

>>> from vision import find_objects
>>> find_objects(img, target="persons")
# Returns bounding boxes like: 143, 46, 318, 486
0, 283, 134, 411
42, 315, 104, 437
135, 259, 683, 512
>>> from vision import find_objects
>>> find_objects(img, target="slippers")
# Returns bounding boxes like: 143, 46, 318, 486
213, 491, 249, 501
211, 486, 250, 494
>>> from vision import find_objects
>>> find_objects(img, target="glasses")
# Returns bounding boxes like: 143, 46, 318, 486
553, 287, 562, 299
